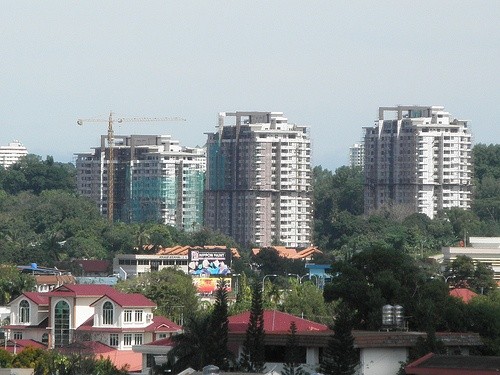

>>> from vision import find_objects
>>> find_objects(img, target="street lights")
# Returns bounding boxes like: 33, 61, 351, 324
263, 274, 278, 292
287, 273, 310, 285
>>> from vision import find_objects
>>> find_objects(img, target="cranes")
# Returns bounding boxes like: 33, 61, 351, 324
75, 113, 189, 224
